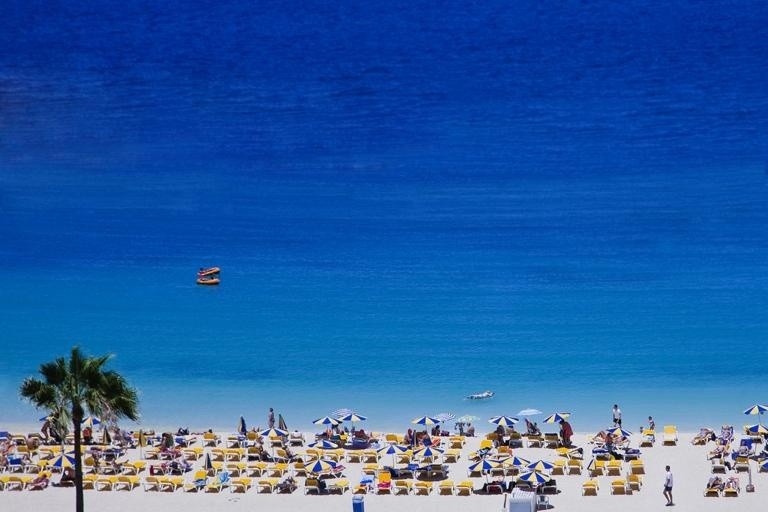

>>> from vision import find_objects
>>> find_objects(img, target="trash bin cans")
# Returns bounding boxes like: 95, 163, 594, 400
509, 487, 537, 512
352, 495, 364, 512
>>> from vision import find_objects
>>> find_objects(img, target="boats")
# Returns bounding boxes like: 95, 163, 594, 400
197, 267, 219, 276
466, 391, 493, 400
197, 278, 219, 284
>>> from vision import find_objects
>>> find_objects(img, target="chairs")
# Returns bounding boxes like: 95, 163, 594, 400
691, 425, 768, 497
0, 425, 677, 494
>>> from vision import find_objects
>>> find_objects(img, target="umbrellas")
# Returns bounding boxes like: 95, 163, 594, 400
81, 416, 101, 425
743, 403, 768, 416
39, 416, 55, 421
47, 447, 77, 467
604, 427, 632, 437
303, 408, 574, 493
234, 414, 247, 433
204, 452, 213, 471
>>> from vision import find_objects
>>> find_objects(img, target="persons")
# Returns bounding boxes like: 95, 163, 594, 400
0, 421, 51, 489
283, 475, 299, 484
467, 390, 494, 399
205, 428, 217, 439
612, 404, 622, 427
248, 407, 306, 461
591, 431, 627, 444
604, 431, 613, 453
61, 467, 76, 481
648, 416, 656, 442
691, 423, 768, 490
82, 424, 194, 470
662, 465, 674, 506
